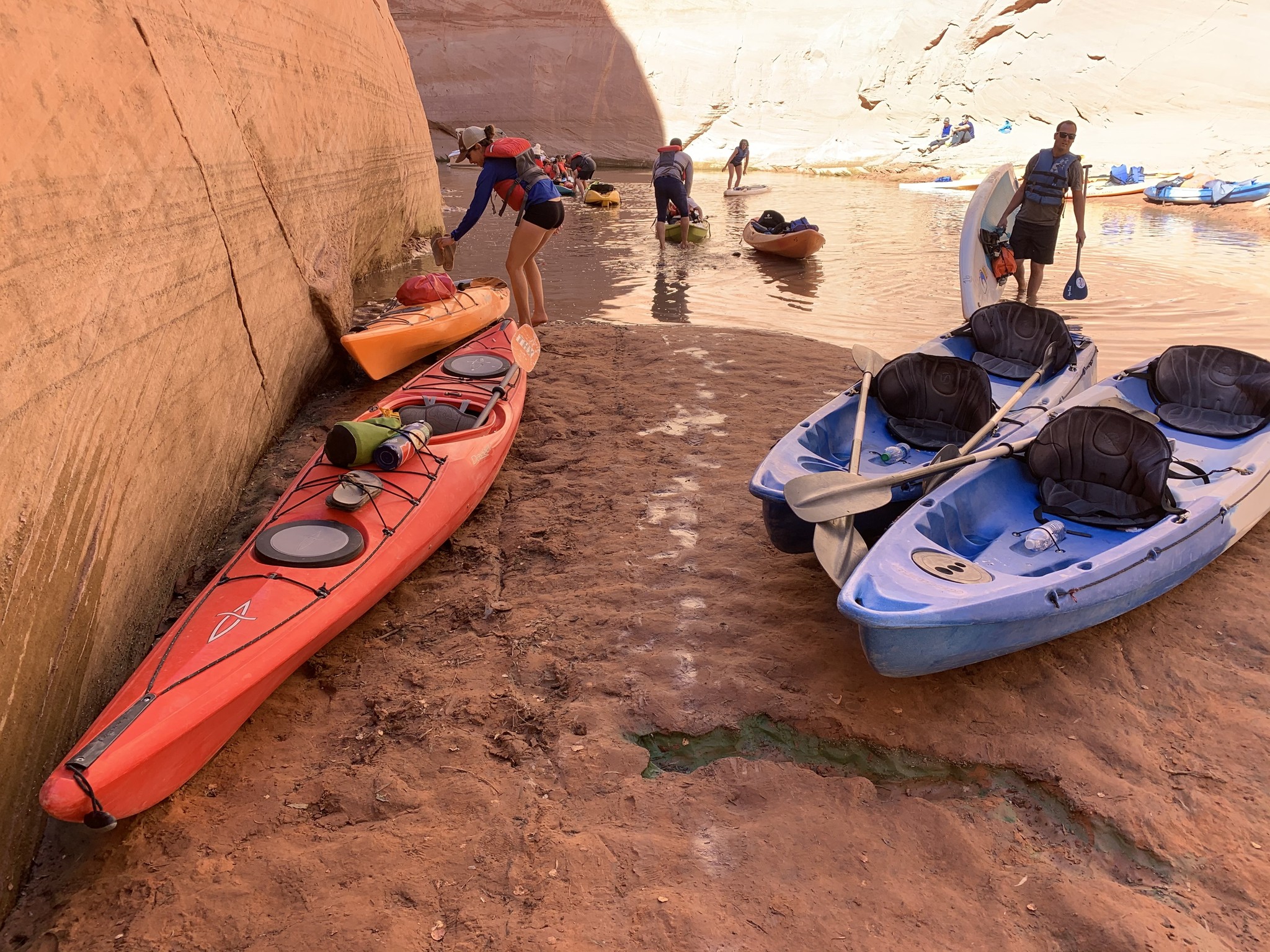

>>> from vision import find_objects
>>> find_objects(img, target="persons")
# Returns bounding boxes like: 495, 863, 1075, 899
438, 125, 565, 330
996, 120, 1086, 296
531, 144, 596, 198
917, 115, 975, 155
653, 138, 693, 251
722, 139, 749, 190
666, 184, 702, 224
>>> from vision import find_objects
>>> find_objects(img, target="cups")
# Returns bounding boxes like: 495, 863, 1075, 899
373, 420, 433, 471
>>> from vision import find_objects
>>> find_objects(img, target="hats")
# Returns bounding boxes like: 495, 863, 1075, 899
550, 157, 556, 162
561, 155, 565, 159
531, 144, 541, 155
455, 126, 486, 163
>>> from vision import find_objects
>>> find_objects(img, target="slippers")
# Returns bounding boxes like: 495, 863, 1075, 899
430, 232, 445, 266
443, 234, 457, 271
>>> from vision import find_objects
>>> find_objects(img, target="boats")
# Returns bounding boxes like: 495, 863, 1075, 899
653, 210, 710, 243
898, 173, 988, 190
1141, 181, 1270, 206
742, 209, 826, 260
747, 300, 1099, 555
583, 181, 621, 209
552, 174, 578, 195
1064, 165, 1196, 200
723, 184, 773, 197
338, 274, 511, 383
36, 312, 528, 829
833, 343, 1270, 680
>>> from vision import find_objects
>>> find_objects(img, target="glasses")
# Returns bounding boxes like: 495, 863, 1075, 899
465, 146, 475, 160
1056, 131, 1076, 139
741, 143, 747, 146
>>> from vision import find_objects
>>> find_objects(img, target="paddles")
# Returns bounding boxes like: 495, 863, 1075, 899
470, 323, 541, 429
1089, 169, 1181, 183
920, 339, 1063, 499
813, 344, 884, 591
783, 397, 1161, 524
455, 276, 508, 292
1063, 163, 1094, 301
676, 215, 718, 225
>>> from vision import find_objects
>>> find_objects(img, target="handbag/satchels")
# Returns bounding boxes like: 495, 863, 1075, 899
990, 241, 1017, 279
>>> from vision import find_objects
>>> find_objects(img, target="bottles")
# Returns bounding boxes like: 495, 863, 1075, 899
1024, 519, 1067, 552
998, 276, 1008, 286
881, 442, 911, 465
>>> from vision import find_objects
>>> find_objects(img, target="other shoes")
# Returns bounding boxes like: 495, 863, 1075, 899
980, 228, 1000, 253
917, 148, 924, 153
951, 143, 958, 147
947, 142, 953, 147
927, 147, 932, 153
